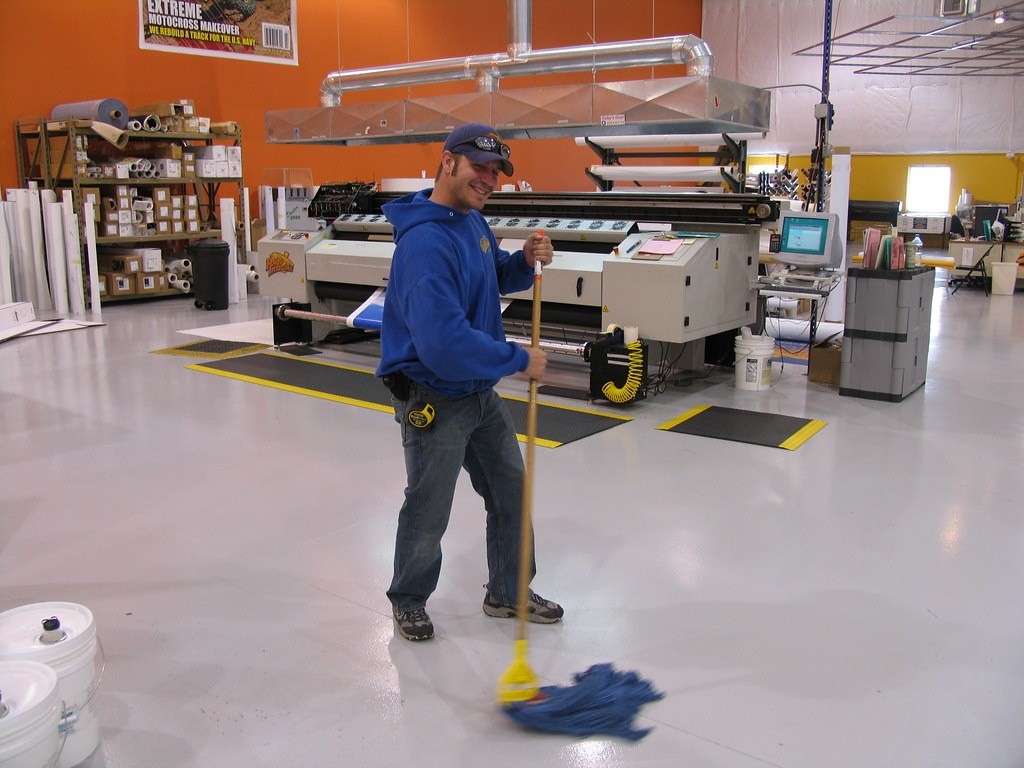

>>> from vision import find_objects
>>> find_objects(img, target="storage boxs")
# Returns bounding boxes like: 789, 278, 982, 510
115, 163, 130, 180
98, 254, 143, 275
809, 330, 844, 386
160, 273, 168, 290
181, 152, 195, 165
194, 160, 215, 179
188, 143, 226, 161
100, 274, 107, 298
47, 183, 204, 239
135, 272, 160, 295
150, 158, 182, 179
185, 117, 198, 133
215, 162, 228, 179
228, 144, 244, 180
178, 99, 197, 115
180, 164, 195, 179
106, 247, 163, 274
158, 144, 181, 158
101, 271, 136, 296
989, 261, 1020, 296
97, 163, 113, 179
198, 114, 213, 132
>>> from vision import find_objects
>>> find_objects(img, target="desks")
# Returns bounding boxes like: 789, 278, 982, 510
945, 234, 1003, 286
897, 212, 952, 251
1001, 241, 1024, 291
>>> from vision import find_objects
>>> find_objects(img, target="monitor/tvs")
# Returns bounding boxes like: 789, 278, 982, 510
775, 211, 843, 276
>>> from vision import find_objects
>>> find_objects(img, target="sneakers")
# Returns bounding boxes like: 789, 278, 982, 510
392, 602, 436, 641
483, 587, 565, 624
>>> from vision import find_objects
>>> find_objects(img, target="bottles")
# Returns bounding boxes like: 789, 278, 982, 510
913, 234, 923, 267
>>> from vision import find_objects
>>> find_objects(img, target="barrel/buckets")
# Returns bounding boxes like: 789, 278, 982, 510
733, 335, 775, 390
0, 601, 105, 768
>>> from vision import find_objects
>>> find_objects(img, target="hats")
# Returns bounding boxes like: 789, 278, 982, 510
442, 123, 515, 177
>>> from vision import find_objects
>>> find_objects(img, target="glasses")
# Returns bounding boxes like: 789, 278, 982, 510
450, 135, 512, 159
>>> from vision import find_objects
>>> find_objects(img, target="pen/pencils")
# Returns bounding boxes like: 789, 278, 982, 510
626, 240, 641, 254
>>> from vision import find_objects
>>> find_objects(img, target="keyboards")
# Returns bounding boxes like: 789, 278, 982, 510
762, 285, 829, 297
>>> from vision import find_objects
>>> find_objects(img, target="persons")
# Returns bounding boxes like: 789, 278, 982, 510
373, 123, 564, 641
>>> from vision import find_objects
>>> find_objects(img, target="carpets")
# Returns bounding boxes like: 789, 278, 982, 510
655, 402, 833, 455
144, 334, 270, 358
186, 351, 637, 450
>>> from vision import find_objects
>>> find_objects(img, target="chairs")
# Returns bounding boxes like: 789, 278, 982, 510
950, 212, 969, 241
947, 239, 996, 300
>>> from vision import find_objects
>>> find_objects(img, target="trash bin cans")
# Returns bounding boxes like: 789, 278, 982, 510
188, 239, 231, 310
990, 262, 1019, 294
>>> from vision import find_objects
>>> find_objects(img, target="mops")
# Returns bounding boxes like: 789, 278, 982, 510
496, 231, 668, 743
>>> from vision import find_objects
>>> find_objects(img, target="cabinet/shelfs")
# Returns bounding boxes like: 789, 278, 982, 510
839, 264, 936, 403
15, 122, 254, 304
1003, 220, 1023, 243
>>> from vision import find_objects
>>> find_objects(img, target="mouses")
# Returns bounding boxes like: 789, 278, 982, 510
815, 271, 832, 278
